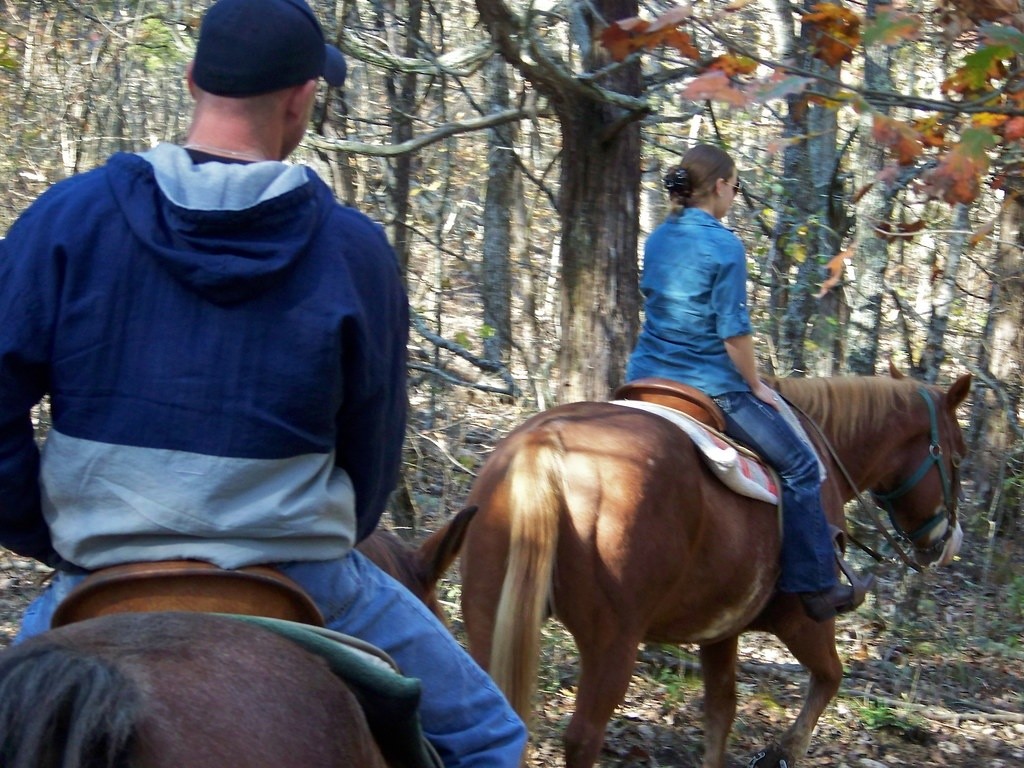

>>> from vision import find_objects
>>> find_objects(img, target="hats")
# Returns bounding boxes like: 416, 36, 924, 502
191, 1, 345, 94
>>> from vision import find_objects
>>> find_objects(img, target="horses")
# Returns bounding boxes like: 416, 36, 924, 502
461, 357, 973, 768
0, 505, 479, 768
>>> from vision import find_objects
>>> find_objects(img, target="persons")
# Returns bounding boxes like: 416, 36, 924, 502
625, 145, 871, 622
0, 2, 530, 768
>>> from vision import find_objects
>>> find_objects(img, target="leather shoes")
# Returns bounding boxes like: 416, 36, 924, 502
804, 568, 874, 621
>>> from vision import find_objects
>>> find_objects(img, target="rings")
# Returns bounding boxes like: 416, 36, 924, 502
774, 398, 777, 401
